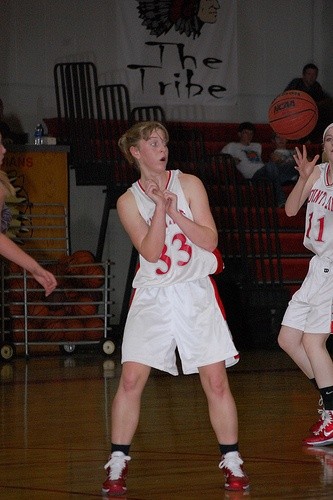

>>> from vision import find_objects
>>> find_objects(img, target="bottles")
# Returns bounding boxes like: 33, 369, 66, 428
34, 124, 42, 146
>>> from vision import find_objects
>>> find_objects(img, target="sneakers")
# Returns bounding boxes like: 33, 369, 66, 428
218, 450, 251, 492
303, 397, 333, 445
100, 449, 133, 496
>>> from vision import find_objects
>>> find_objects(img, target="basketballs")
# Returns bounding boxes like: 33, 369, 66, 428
268, 88, 317, 141
8, 249, 106, 342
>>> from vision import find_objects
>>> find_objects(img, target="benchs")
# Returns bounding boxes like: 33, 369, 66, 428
71, 123, 317, 324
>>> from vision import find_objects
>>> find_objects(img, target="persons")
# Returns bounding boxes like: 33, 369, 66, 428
101, 120, 251, 500
277, 121, 332, 448
214, 63, 333, 209
0, 138, 58, 379
